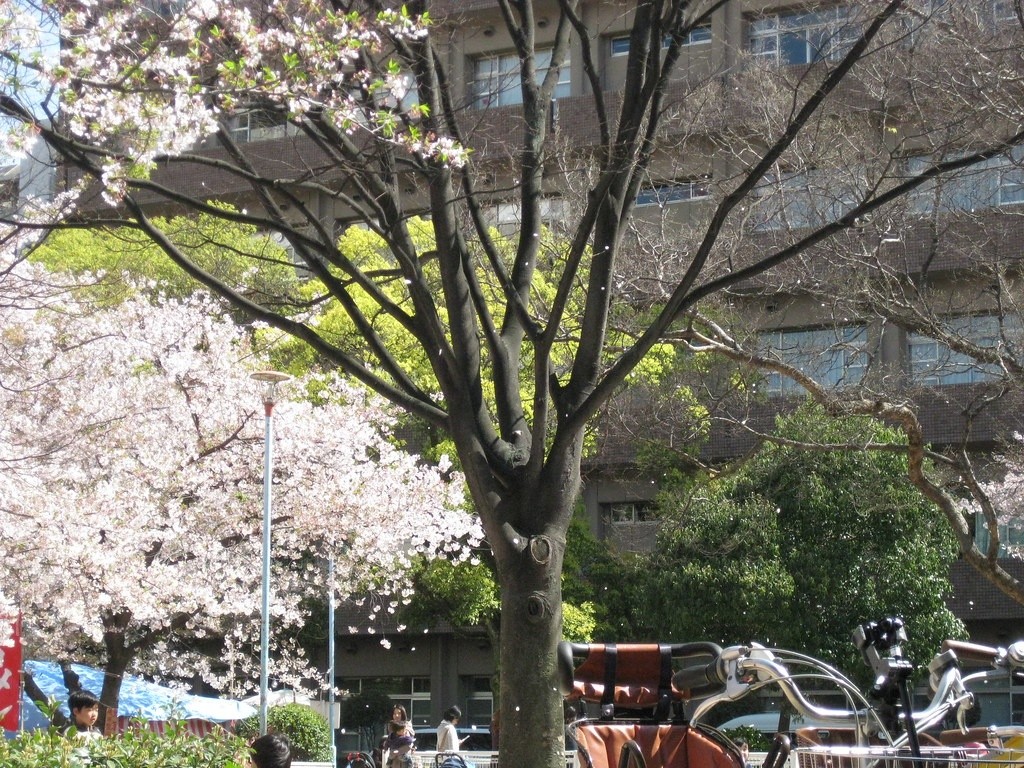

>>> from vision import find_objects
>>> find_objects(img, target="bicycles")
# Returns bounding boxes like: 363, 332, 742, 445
796, 638, 1024, 767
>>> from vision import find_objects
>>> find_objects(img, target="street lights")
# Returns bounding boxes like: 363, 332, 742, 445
247, 371, 292, 743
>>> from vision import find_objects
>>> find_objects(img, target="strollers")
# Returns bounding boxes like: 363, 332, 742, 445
434, 752, 476, 768
348, 752, 378, 768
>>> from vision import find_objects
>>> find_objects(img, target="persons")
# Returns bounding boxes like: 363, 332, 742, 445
60, 689, 105, 742
435, 705, 464, 763
248, 732, 292, 767
346, 702, 420, 768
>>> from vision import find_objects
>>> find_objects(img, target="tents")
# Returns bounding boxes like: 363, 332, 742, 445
4, 658, 260, 750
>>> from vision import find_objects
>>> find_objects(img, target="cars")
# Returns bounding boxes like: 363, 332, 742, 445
410, 726, 493, 752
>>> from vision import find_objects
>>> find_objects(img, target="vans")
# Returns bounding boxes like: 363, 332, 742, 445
713, 711, 864, 751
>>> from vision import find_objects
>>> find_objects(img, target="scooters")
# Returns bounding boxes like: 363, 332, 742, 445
555, 619, 1024, 768
